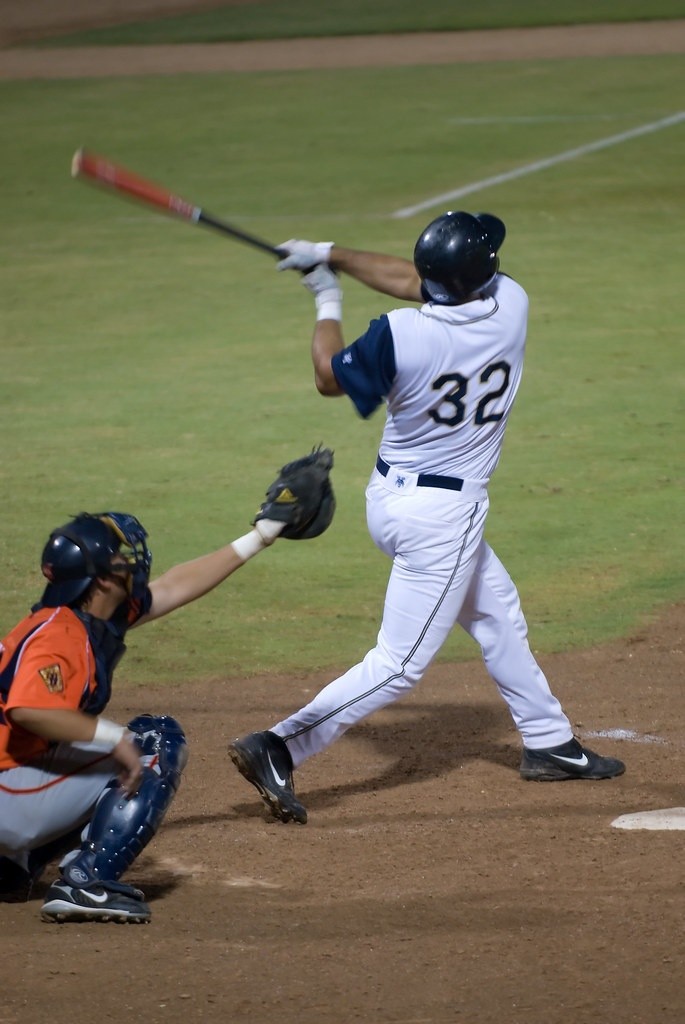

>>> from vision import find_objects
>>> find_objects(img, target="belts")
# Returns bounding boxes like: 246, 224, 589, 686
374, 455, 463, 494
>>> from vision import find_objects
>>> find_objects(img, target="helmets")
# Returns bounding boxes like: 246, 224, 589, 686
413, 210, 506, 304
41, 512, 126, 611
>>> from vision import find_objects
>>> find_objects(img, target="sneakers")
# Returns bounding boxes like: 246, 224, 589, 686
226, 729, 308, 824
517, 736, 625, 782
39, 878, 152, 924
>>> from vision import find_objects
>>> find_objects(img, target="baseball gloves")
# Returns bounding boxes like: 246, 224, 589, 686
249, 441, 337, 541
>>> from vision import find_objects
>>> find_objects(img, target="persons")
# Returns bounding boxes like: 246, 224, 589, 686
0, 445, 335, 924
224, 211, 625, 823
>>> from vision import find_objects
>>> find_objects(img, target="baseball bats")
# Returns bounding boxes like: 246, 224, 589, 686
71, 147, 340, 278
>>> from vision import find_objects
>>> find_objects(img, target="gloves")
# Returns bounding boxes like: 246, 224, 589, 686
301, 263, 351, 322
274, 238, 336, 272
